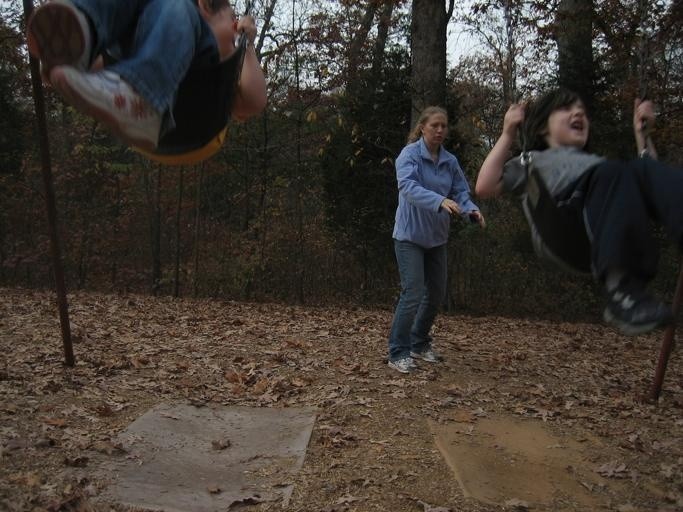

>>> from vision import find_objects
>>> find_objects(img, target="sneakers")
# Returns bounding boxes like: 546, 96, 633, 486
387, 357, 417, 373
25, 0, 91, 89
48, 63, 165, 154
410, 345, 441, 363
600, 271, 672, 336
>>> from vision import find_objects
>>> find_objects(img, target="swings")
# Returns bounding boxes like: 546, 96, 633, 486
505, 0, 658, 278
129, 0, 255, 165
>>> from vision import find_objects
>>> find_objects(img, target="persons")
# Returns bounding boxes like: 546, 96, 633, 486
388, 106, 486, 374
475, 88, 682, 337
29, 2, 268, 156
